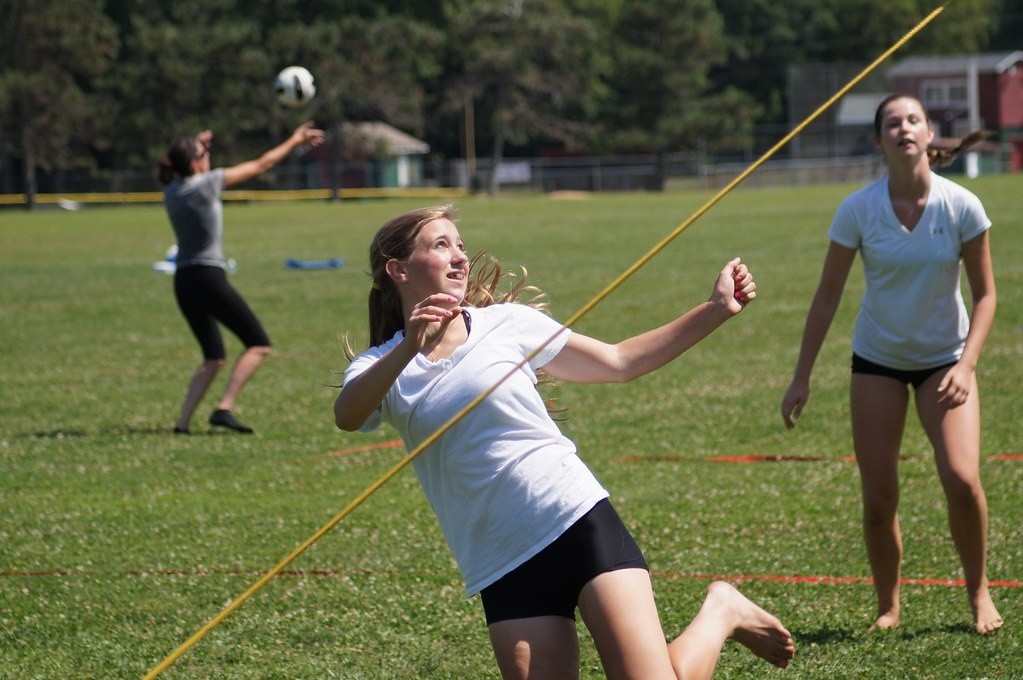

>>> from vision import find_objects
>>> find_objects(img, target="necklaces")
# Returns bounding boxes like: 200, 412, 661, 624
425, 349, 437, 363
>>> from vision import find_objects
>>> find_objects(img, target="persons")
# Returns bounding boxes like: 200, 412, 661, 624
334, 205, 795, 679
781, 93, 1005, 636
159, 121, 326, 435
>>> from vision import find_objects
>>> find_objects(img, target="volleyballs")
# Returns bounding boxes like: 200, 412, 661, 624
272, 65, 316, 108
